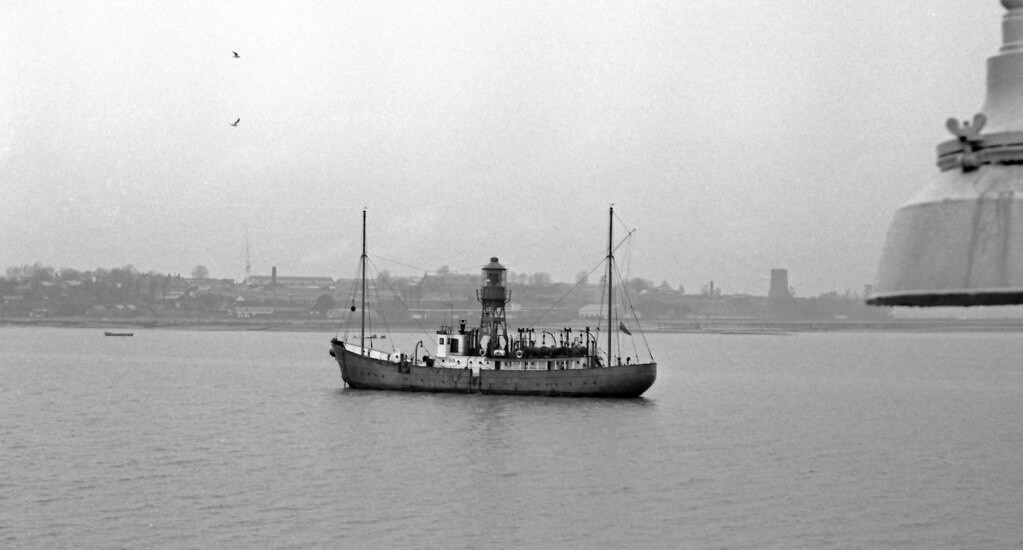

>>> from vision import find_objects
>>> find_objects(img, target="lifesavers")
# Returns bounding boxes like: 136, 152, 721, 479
479, 349, 486, 356
516, 350, 523, 358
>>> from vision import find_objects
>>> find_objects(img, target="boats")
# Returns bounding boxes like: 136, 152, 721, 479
329, 202, 658, 398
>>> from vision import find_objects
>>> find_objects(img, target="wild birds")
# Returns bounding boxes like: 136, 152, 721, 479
232, 50, 240, 58
230, 118, 240, 126
